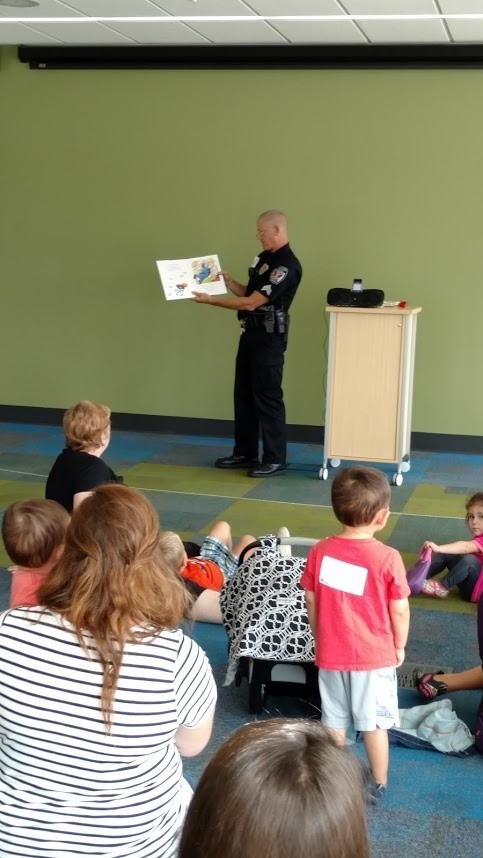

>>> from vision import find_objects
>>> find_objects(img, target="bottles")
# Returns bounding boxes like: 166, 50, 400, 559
352, 279, 362, 291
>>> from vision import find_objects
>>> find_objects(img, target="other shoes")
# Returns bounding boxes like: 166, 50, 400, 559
359, 767, 384, 804
412, 670, 447, 699
421, 578, 449, 599
275, 527, 291, 557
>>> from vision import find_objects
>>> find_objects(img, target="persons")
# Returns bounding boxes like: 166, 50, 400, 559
192, 209, 302, 478
0, 401, 483, 858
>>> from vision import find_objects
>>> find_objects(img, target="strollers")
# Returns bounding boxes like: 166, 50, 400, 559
221, 536, 323, 714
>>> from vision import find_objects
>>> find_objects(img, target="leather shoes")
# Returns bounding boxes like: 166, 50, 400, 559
247, 462, 287, 477
215, 454, 260, 468
